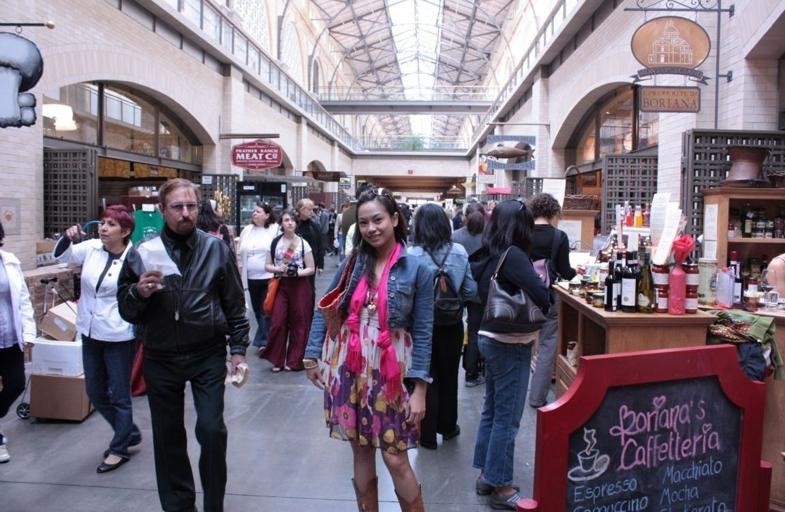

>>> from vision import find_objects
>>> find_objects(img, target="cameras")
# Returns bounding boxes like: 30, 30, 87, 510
287, 263, 297, 278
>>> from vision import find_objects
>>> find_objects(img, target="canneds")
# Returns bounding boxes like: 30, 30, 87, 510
727, 223, 734, 237
751, 208, 785, 239
568, 267, 609, 308
650, 287, 670, 314
652, 262, 670, 288
683, 288, 698, 314
686, 263, 699, 287
748, 277, 758, 298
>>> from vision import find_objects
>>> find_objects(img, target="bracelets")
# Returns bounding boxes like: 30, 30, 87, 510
302, 357, 319, 369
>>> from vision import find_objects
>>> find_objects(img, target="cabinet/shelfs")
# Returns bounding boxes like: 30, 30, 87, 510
551, 284, 719, 402
700, 184, 785, 306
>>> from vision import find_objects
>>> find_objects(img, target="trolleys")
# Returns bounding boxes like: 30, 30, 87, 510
16, 276, 58, 418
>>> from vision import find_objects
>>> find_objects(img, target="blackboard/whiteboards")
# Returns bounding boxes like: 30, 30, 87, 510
531, 344, 767, 512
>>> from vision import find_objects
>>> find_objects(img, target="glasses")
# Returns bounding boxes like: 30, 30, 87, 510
263, 202, 269, 215
163, 202, 200, 211
510, 199, 527, 212
367, 187, 396, 211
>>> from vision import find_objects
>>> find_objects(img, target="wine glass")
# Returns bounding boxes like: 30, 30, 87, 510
760, 268, 777, 312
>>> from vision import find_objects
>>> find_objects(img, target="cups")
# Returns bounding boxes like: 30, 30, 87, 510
569, 274, 605, 307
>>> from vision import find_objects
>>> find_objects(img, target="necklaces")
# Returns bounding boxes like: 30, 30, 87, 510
172, 276, 181, 321
367, 284, 378, 315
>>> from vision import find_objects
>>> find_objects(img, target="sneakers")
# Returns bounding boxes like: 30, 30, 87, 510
421, 434, 437, 449
466, 376, 485, 387
259, 345, 265, 352
273, 365, 284, 372
0, 445, 9, 462
476, 477, 520, 495
285, 366, 292, 371
442, 425, 459, 440
491, 491, 538, 510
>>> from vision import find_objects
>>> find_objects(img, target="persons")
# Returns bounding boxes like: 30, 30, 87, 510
116, 177, 251, 511
0, 220, 37, 464
52, 205, 143, 474
468, 200, 552, 511
405, 202, 482, 450
196, 182, 607, 389
523, 193, 588, 408
303, 187, 436, 511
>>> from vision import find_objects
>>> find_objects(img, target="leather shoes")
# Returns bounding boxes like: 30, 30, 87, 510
97, 455, 129, 472
104, 434, 141, 458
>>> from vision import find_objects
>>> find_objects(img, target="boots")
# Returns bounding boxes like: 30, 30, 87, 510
393, 483, 424, 512
351, 476, 378, 512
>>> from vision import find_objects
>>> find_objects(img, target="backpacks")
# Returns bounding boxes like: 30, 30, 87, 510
532, 226, 560, 291
424, 244, 463, 325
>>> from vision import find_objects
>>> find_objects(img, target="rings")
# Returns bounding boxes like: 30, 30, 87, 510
69, 230, 73, 234
147, 282, 152, 289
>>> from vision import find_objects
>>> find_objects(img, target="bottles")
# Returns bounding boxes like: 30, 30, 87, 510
729, 250, 743, 307
760, 253, 770, 274
604, 252, 654, 314
624, 200, 651, 226
742, 202, 753, 238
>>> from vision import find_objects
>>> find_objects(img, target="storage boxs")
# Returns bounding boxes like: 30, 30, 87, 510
27, 240, 97, 422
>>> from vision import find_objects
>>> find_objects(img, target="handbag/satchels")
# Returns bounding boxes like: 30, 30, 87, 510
263, 278, 279, 314
483, 245, 546, 332
317, 253, 356, 341
208, 224, 236, 259
